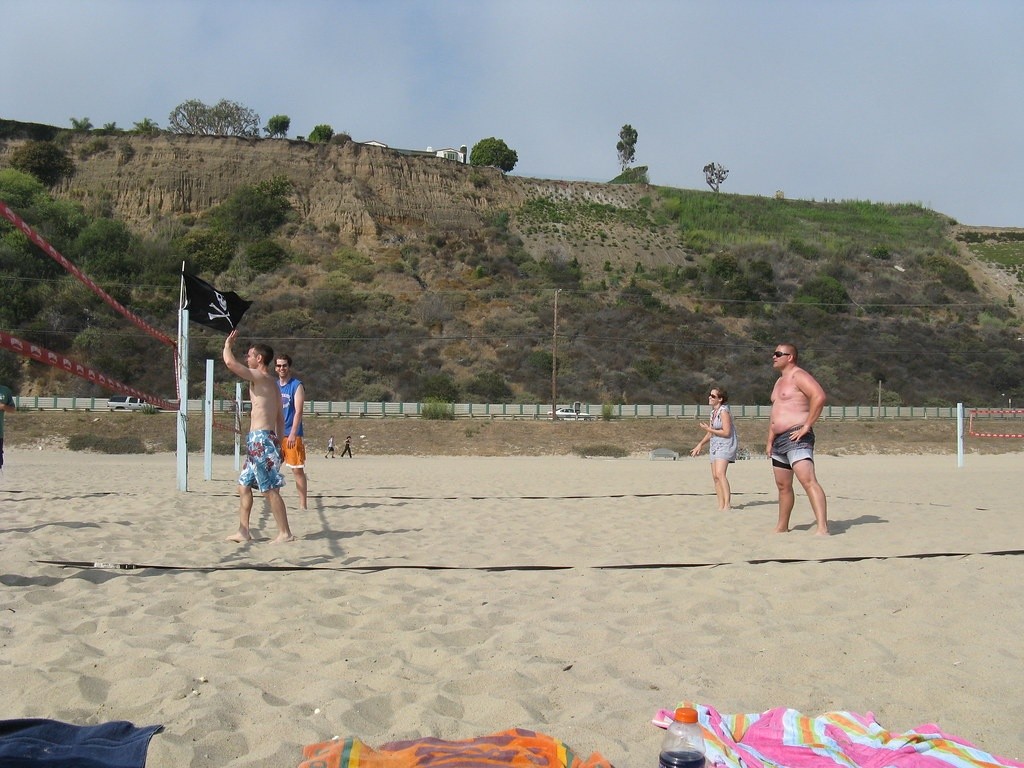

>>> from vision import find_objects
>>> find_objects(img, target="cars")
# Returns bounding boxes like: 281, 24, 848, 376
547, 408, 590, 420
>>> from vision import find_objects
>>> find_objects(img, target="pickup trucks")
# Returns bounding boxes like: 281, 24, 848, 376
107, 395, 162, 412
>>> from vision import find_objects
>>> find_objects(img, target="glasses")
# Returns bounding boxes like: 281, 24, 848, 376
275, 364, 287, 367
774, 352, 794, 359
709, 394, 720, 399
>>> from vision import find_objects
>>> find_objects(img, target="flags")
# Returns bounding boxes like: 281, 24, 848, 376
180, 271, 253, 334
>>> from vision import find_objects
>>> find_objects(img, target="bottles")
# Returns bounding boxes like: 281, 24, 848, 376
658, 708, 706, 768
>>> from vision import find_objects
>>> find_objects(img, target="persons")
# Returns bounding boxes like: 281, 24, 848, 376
0, 385, 15, 470
324, 435, 335, 458
689, 387, 738, 510
222, 329, 293, 543
765, 343, 828, 536
271, 354, 307, 510
340, 436, 352, 458
